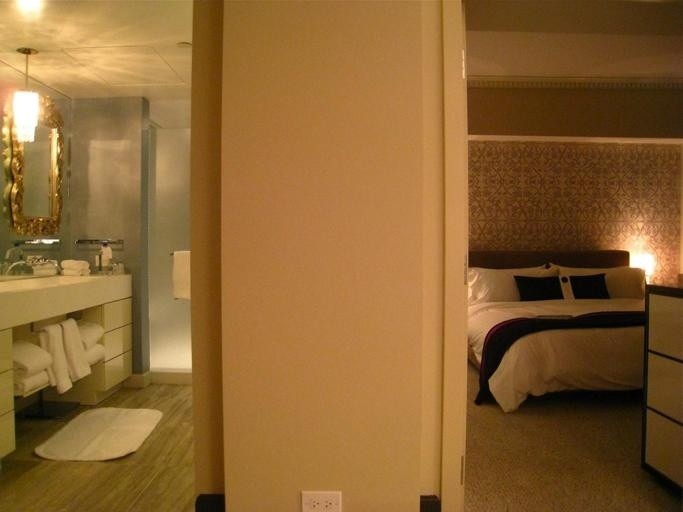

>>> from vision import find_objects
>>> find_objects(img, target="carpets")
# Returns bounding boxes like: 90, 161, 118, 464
33, 407, 163, 462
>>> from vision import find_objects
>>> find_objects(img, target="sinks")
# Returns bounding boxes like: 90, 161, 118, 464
0, 275, 52, 283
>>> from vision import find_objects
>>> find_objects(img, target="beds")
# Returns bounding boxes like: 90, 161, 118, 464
468, 248, 651, 414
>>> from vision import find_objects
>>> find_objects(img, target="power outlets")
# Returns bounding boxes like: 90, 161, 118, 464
298, 486, 340, 510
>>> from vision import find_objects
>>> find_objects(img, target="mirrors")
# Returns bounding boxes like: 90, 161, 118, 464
0, 89, 66, 240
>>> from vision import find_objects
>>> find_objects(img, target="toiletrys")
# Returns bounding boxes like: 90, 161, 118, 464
101, 240, 113, 271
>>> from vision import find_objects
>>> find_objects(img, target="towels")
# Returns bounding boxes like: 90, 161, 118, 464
62, 269, 92, 276
60, 318, 91, 382
37, 323, 72, 393
171, 249, 190, 298
12, 371, 51, 398
85, 342, 107, 368
57, 259, 90, 271
75, 319, 104, 351
10, 339, 53, 377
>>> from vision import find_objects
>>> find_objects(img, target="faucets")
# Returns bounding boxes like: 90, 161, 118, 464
3, 236, 60, 276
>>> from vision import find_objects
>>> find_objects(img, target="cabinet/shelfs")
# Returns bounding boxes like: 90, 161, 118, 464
638, 280, 683, 493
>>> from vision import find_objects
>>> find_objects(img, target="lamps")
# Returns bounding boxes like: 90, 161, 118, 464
8, 46, 42, 144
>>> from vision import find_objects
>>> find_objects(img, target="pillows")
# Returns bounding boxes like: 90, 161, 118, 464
470, 262, 646, 306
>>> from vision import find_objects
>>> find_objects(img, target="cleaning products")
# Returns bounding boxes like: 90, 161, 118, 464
107, 259, 117, 275
